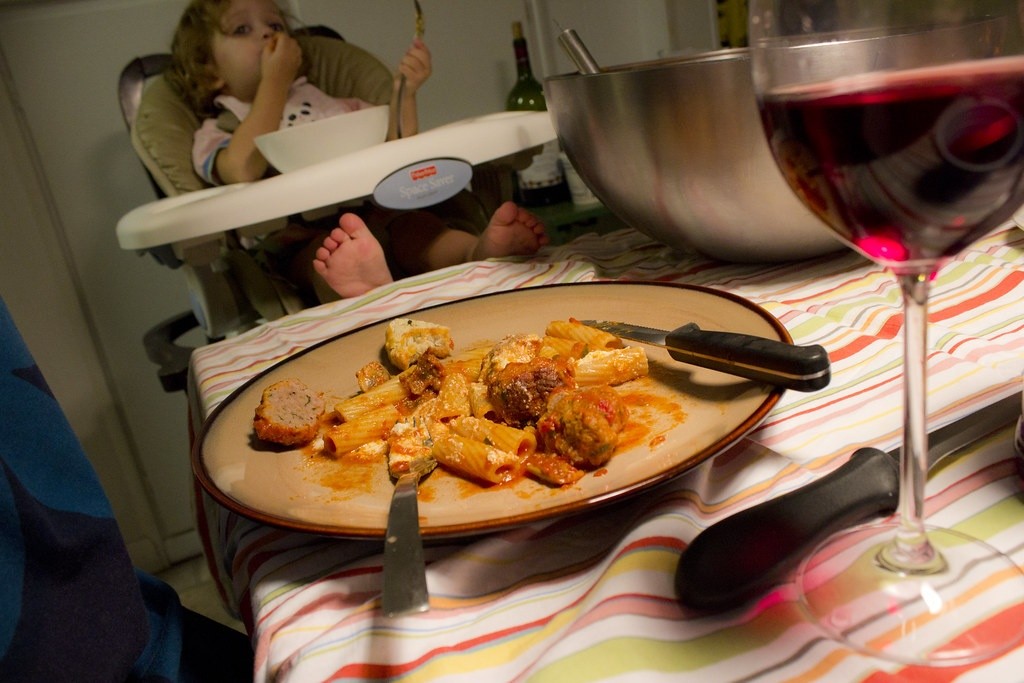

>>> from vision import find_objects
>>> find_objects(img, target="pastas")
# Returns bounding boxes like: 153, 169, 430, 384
320, 320, 650, 484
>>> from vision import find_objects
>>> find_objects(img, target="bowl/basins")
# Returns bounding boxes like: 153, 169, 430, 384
544, 16, 1006, 263
253, 103, 388, 175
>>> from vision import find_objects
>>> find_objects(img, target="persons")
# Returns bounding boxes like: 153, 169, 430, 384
0, 295, 256, 683
160, 0, 551, 299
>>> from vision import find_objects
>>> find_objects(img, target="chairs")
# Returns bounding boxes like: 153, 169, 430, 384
116, 25, 557, 393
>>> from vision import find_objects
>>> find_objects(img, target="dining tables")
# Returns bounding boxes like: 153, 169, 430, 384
188, 204, 1024, 682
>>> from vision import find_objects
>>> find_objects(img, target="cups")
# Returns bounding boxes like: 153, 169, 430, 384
559, 153, 601, 204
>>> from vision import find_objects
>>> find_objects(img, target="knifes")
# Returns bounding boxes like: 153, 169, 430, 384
579, 319, 833, 392
675, 389, 1023, 610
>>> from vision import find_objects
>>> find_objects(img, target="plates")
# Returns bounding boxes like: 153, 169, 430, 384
191, 280, 792, 536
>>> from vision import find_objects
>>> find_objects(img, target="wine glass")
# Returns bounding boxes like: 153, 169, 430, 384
748, 0, 1024, 669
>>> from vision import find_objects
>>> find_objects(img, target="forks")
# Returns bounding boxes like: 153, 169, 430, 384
379, 416, 435, 617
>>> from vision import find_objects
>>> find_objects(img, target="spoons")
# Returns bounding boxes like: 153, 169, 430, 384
396, 1, 425, 139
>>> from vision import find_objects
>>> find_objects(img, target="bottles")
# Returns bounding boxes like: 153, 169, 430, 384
508, 20, 568, 207
